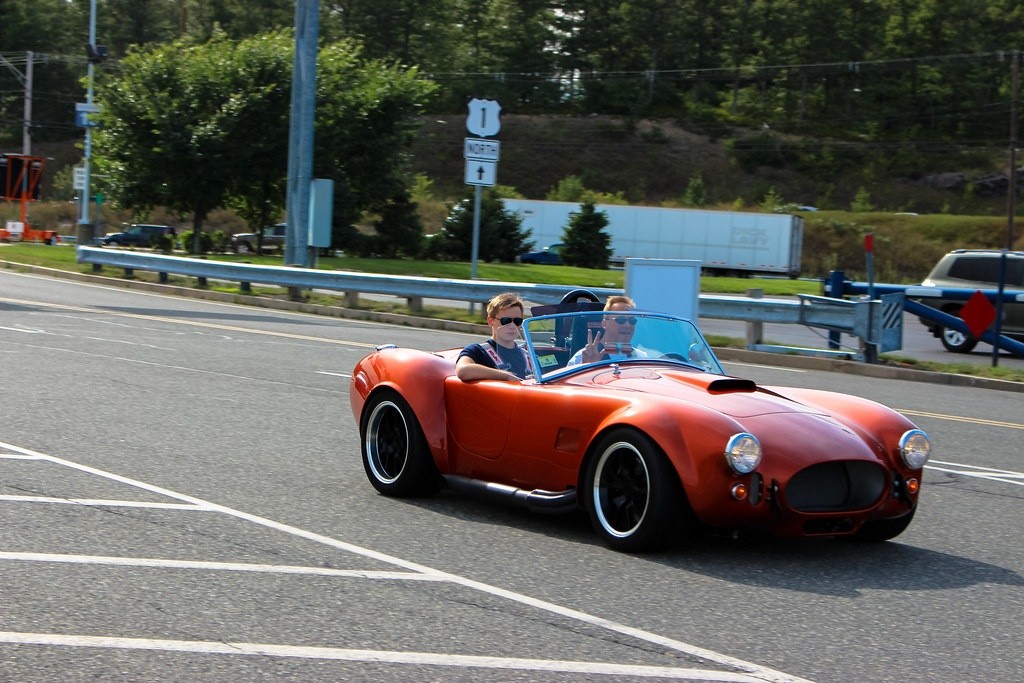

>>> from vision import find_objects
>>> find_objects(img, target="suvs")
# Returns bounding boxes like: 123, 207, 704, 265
916, 247, 1024, 351
103, 221, 176, 252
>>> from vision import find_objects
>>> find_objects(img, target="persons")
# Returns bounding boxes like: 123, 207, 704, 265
566, 294, 650, 367
455, 291, 535, 382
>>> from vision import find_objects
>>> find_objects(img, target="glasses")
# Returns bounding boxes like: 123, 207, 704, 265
491, 315, 523, 327
604, 317, 637, 325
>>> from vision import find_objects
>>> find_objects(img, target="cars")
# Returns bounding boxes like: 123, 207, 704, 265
230, 221, 309, 257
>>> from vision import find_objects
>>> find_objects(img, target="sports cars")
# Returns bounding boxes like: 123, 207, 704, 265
345, 290, 932, 559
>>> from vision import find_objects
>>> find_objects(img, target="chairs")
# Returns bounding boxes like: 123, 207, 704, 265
567, 309, 605, 359
